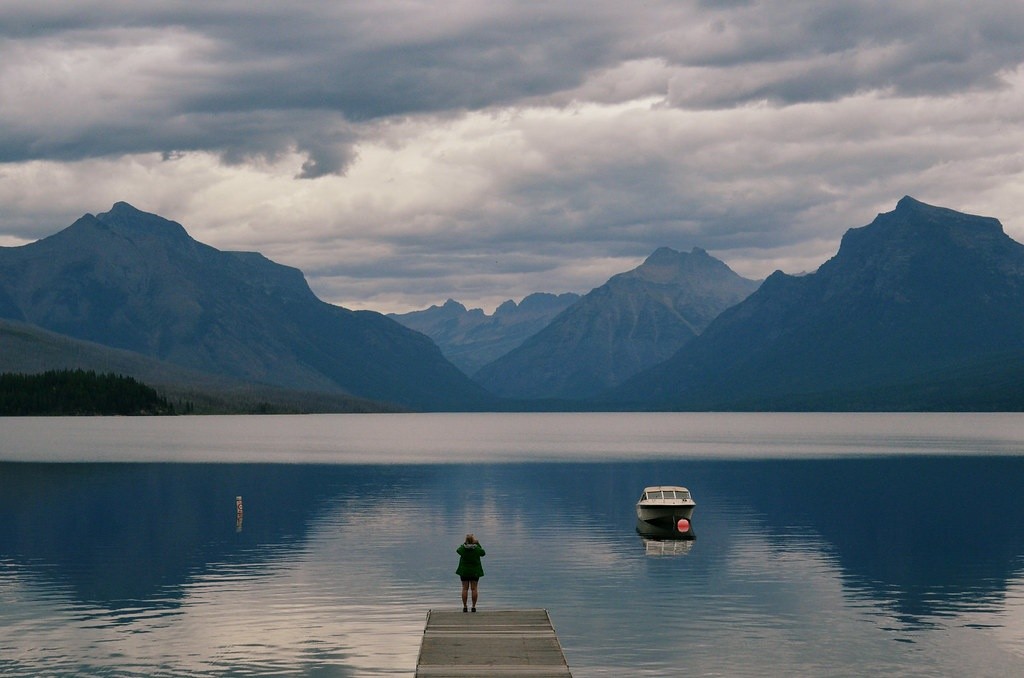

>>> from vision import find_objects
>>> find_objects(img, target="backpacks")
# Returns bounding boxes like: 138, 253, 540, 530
462, 545, 478, 568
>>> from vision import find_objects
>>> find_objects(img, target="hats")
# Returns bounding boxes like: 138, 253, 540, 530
466, 533, 476, 544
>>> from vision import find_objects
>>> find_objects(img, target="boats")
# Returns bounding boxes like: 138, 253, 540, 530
635, 485, 697, 524
636, 518, 697, 558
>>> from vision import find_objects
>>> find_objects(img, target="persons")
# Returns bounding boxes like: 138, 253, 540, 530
456, 533, 485, 612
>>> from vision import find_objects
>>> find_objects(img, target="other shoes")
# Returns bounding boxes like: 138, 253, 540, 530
472, 608, 476, 612
463, 608, 467, 612
